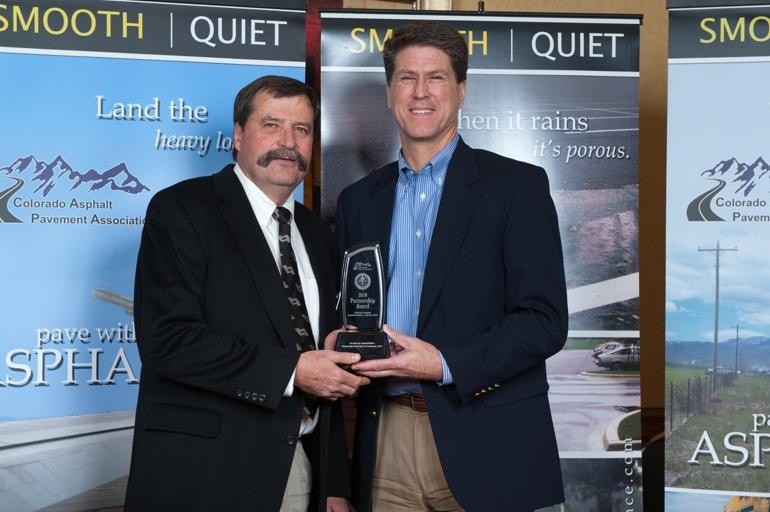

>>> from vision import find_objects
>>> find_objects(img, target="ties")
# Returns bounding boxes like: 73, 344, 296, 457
272, 205, 319, 422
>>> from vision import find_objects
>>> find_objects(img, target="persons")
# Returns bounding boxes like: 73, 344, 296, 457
123, 72, 374, 510
321, 21, 573, 511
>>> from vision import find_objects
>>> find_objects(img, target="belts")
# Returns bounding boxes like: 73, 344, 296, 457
390, 392, 427, 411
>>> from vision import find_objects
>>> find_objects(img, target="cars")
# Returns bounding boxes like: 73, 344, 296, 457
703, 365, 769, 378
591, 340, 641, 376
